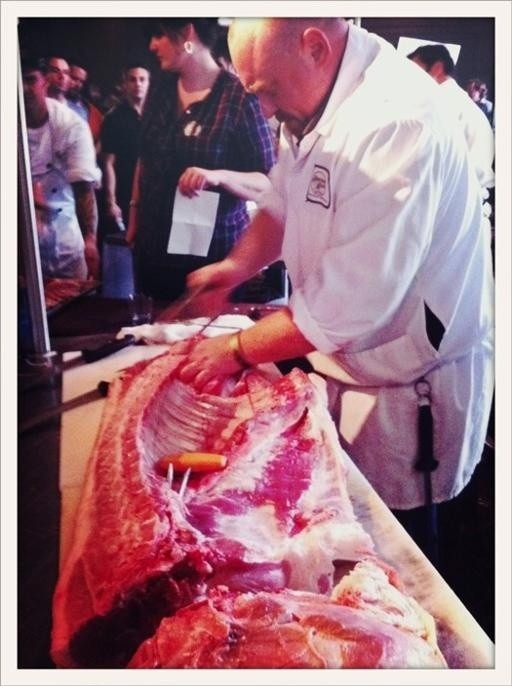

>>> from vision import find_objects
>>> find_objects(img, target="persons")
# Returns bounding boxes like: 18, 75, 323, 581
154, 19, 494, 544
18, 18, 496, 321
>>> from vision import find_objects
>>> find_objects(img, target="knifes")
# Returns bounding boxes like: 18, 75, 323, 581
25, 334, 135, 392
19, 380, 110, 430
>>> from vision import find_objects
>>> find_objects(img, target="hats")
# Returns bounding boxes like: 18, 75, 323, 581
22, 52, 47, 73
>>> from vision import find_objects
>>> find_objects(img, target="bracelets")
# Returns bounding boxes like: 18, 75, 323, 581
225, 325, 255, 373
